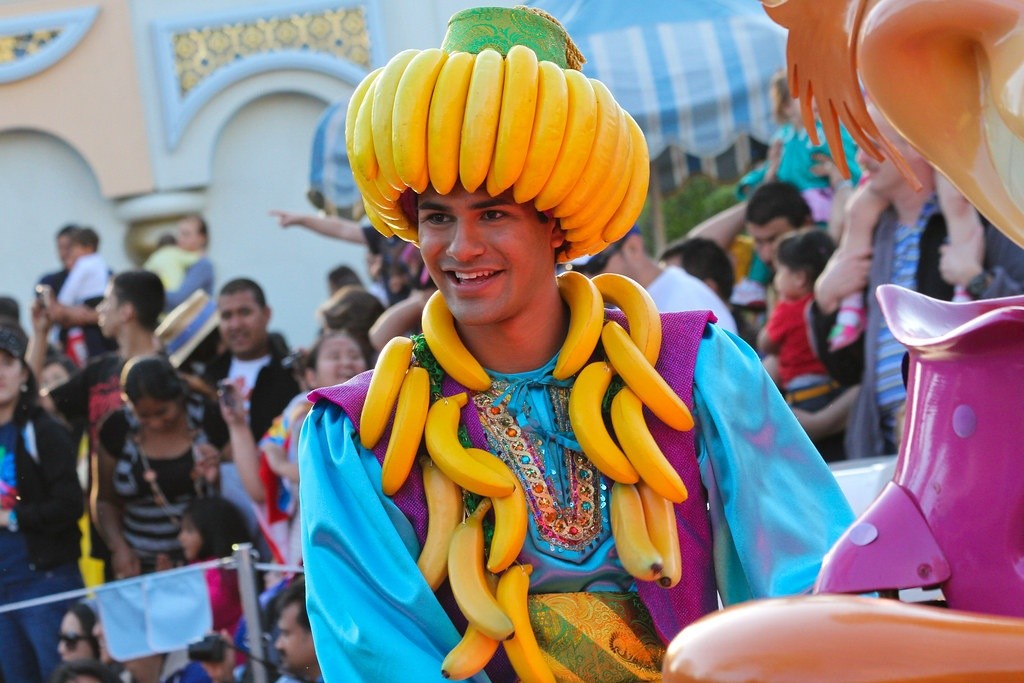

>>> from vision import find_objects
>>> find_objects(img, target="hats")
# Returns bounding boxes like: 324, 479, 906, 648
343, 5, 650, 263
0, 326, 28, 356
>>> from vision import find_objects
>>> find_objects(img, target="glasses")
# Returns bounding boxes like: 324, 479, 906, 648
57, 631, 90, 651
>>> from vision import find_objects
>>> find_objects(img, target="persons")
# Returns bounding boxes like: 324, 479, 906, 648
298, 8, 881, 683
556, 225, 737, 334
0, 209, 440, 683
660, 71, 1024, 462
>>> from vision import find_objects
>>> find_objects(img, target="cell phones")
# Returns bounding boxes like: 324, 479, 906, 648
35, 286, 50, 310
217, 382, 237, 408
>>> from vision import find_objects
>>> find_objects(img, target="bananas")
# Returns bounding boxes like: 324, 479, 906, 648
360, 270, 694, 683
345, 43, 649, 262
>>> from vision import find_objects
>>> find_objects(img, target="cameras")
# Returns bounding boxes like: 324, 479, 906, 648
187, 635, 226, 662
